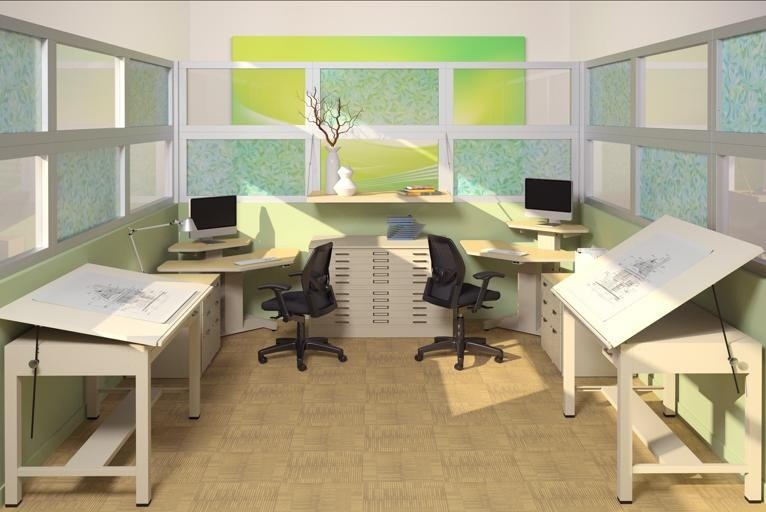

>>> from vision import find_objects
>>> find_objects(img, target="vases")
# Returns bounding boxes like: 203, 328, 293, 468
334, 164, 356, 197
324, 145, 341, 195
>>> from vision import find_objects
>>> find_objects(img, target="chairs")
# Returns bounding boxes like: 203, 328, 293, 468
257, 242, 347, 370
416, 234, 504, 370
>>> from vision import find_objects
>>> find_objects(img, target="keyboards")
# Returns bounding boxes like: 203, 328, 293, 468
487, 248, 529, 256
234, 257, 272, 266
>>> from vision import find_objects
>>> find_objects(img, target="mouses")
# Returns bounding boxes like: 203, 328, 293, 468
272, 258, 280, 261
480, 249, 488, 254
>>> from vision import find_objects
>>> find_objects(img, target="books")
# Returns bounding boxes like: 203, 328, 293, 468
398, 185, 442, 197
480, 248, 529, 258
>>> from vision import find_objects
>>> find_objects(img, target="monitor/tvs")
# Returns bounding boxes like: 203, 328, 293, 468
524, 177, 573, 227
189, 194, 237, 245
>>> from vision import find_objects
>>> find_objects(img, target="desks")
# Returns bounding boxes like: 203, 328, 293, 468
3, 286, 214, 506
460, 220, 590, 337
560, 288, 762, 505
157, 239, 301, 337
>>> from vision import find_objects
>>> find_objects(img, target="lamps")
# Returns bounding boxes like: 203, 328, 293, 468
128, 218, 198, 271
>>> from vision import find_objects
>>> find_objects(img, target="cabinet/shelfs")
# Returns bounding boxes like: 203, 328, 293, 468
540, 271, 637, 377
304, 237, 460, 338
145, 273, 221, 378
